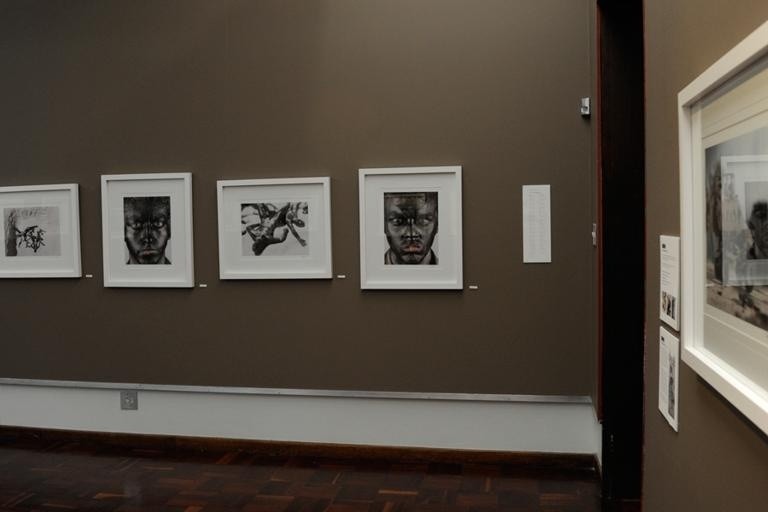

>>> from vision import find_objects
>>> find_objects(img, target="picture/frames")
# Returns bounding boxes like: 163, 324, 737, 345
1, 181, 84, 281
98, 170, 199, 291
354, 165, 467, 292
675, 18, 767, 440
212, 175, 338, 282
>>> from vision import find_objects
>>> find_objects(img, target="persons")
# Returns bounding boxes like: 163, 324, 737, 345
123, 196, 173, 266
240, 202, 307, 256
383, 194, 440, 265
